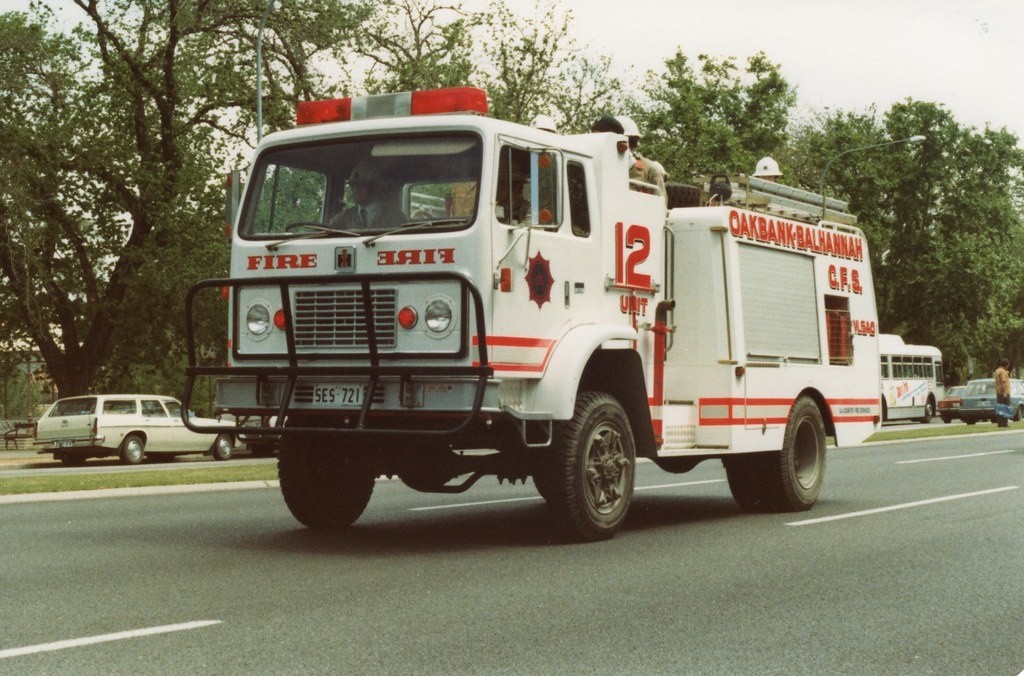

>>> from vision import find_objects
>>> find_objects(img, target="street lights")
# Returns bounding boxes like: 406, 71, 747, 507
820, 132, 925, 224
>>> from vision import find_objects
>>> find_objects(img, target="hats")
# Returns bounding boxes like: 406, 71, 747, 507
592, 114, 624, 133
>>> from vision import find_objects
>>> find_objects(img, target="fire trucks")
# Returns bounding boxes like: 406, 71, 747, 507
181, 87, 888, 539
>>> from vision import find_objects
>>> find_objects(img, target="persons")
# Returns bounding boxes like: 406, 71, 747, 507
751, 156, 785, 183
589, 114, 668, 206
995, 358, 1010, 427
325, 160, 408, 229
481, 156, 544, 232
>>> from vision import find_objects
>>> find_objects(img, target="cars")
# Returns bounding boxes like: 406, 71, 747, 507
33, 394, 250, 466
938, 385, 977, 423
959, 377, 1024, 426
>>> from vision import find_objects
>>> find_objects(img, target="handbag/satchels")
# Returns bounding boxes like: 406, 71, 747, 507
995, 397, 1017, 419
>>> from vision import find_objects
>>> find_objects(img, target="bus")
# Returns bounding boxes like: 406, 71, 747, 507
876, 334, 945, 423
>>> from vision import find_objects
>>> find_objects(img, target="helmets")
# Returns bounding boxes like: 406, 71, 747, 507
752, 157, 783, 177
614, 116, 642, 139
530, 114, 557, 133
498, 157, 529, 184
345, 159, 377, 182
